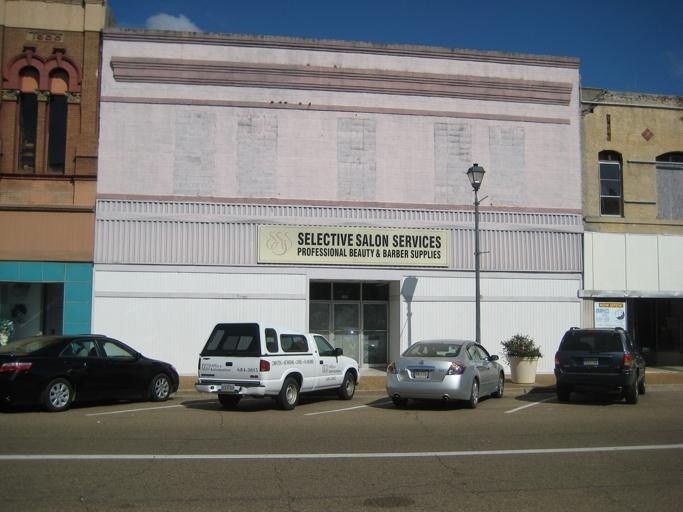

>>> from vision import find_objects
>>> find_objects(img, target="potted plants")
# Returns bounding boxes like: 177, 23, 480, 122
501, 334, 542, 383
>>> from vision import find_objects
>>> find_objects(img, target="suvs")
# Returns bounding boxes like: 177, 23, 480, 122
552, 323, 648, 406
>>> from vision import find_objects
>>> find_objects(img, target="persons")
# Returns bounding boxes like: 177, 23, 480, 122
371, 311, 385, 346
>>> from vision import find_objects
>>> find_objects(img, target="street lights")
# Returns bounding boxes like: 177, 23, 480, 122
465, 161, 487, 343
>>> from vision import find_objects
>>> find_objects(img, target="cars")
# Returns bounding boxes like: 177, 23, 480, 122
381, 337, 505, 409
316, 327, 359, 334
2, 331, 181, 413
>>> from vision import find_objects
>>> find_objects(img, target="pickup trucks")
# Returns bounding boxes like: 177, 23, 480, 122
359, 328, 387, 361
191, 317, 363, 412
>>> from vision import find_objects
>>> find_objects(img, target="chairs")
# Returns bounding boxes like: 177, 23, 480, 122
62, 344, 88, 356
